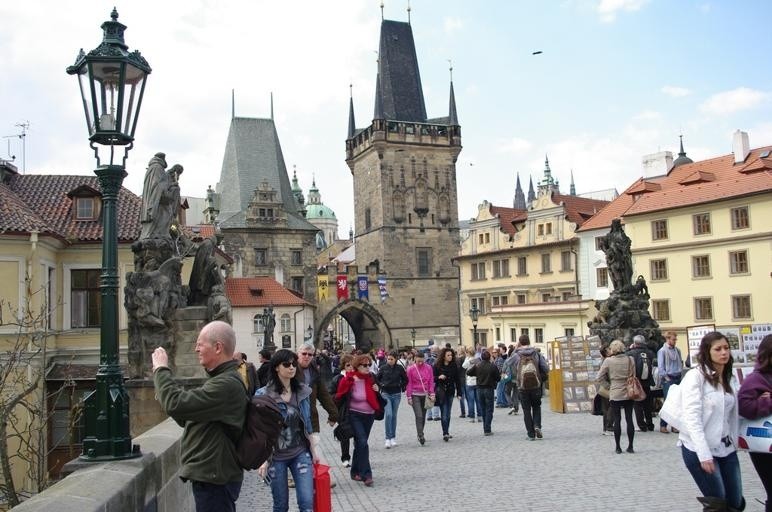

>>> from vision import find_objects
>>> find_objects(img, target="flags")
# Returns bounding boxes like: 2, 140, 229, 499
336, 275, 349, 305
377, 275, 389, 306
358, 276, 369, 304
317, 274, 329, 304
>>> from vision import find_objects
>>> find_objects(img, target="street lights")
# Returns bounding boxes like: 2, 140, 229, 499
64, 0, 160, 466
468, 303, 481, 349
306, 323, 313, 338
411, 325, 417, 350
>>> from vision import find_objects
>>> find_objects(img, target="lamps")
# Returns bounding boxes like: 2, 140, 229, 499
305, 324, 314, 341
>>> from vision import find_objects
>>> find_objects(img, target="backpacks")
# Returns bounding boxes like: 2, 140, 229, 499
636, 348, 652, 381
218, 374, 284, 472
515, 351, 543, 392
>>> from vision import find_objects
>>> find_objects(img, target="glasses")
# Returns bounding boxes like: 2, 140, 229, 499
301, 352, 314, 357
282, 361, 299, 368
346, 363, 351, 367
360, 363, 372, 368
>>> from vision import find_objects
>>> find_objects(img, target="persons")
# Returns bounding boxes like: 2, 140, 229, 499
626, 334, 656, 432
739, 333, 772, 512
596, 338, 637, 453
594, 345, 615, 437
678, 330, 749, 512
657, 330, 684, 433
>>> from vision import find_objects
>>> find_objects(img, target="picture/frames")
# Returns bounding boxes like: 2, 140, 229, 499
545, 332, 609, 415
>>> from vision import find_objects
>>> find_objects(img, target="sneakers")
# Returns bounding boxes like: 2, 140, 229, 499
535, 426, 544, 439
601, 430, 615, 436
427, 418, 433, 421
417, 432, 426, 446
384, 438, 391, 449
434, 417, 442, 422
342, 457, 352, 468
508, 407, 520, 416
615, 447, 635, 454
389, 436, 398, 447
637, 425, 680, 435
443, 433, 453, 442
459, 414, 483, 424
351, 472, 363, 482
525, 432, 536, 441
483, 431, 495, 436
363, 472, 375, 486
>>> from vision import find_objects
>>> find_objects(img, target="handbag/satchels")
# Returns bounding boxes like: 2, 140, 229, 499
312, 463, 331, 512
657, 383, 716, 437
423, 395, 437, 409
626, 376, 647, 402
734, 411, 772, 455
